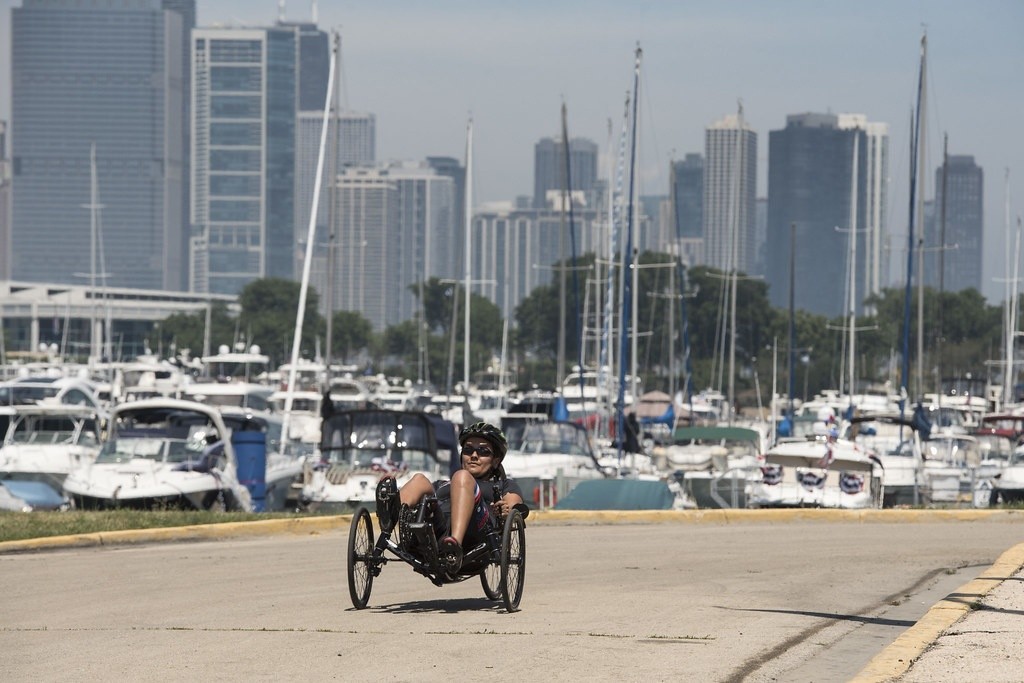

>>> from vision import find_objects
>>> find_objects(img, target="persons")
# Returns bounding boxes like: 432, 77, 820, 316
376, 422, 524, 575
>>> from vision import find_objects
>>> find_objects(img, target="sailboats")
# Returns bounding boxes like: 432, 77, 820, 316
0, 19, 1023, 512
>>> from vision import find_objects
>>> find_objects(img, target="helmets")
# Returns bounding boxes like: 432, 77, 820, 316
458, 423, 507, 457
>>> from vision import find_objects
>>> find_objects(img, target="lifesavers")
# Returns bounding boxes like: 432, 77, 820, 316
533, 485, 559, 505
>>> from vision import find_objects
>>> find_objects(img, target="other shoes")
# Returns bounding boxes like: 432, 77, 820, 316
438, 536, 462, 574
376, 474, 401, 529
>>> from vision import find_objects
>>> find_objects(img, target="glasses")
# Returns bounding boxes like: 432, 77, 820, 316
462, 446, 494, 457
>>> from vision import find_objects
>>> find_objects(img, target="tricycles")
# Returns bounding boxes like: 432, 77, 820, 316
346, 476, 528, 614
